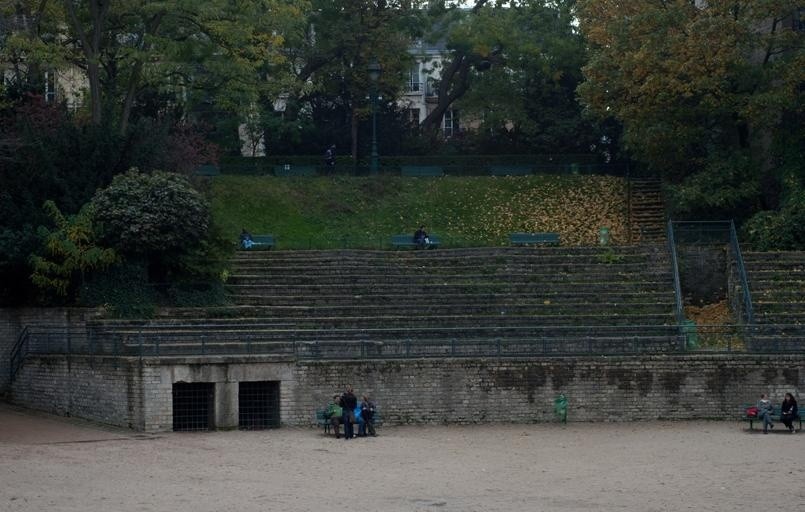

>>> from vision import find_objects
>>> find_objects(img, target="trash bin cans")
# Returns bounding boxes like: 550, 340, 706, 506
684, 320, 697, 348
599, 227, 609, 246
555, 397, 568, 424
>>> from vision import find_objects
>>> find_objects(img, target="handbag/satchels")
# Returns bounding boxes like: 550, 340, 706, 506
746, 407, 758, 416
349, 411, 355, 424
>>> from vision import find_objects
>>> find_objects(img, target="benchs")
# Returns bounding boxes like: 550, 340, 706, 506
391, 233, 442, 250
738, 400, 804, 430
238, 234, 275, 250
509, 231, 563, 248
314, 405, 381, 439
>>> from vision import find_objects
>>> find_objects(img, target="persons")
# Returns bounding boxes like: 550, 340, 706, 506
361, 395, 376, 438
415, 226, 430, 249
240, 227, 255, 252
780, 391, 798, 434
339, 386, 358, 439
757, 392, 775, 435
328, 394, 343, 439
